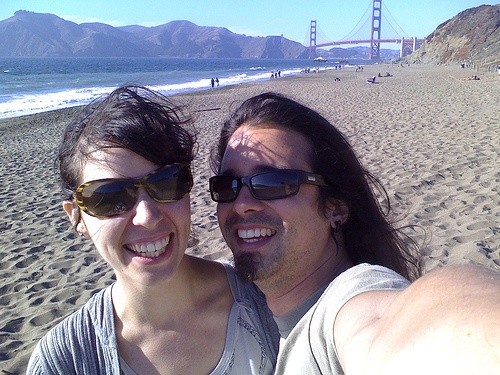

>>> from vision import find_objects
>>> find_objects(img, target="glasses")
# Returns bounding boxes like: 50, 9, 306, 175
209, 168, 327, 203
70, 162, 194, 218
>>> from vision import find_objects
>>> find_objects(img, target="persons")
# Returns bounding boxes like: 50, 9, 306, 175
209, 90, 500, 375
25, 80, 280, 375
378, 71, 392, 78
304, 64, 364, 74
270, 71, 281, 78
